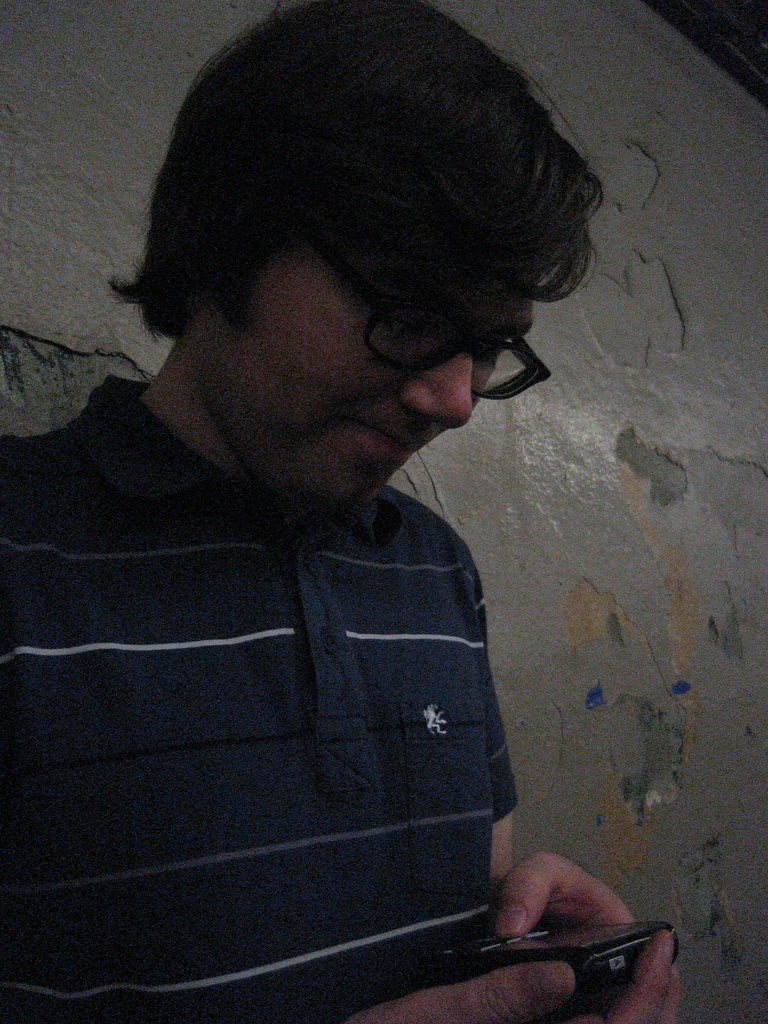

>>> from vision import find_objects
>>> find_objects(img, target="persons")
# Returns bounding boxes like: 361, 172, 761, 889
1, 0, 682, 1023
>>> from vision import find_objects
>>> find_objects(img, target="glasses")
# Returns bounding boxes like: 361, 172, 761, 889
303, 220, 551, 400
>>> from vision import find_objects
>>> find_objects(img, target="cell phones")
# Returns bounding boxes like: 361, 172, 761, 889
446, 920, 679, 998
